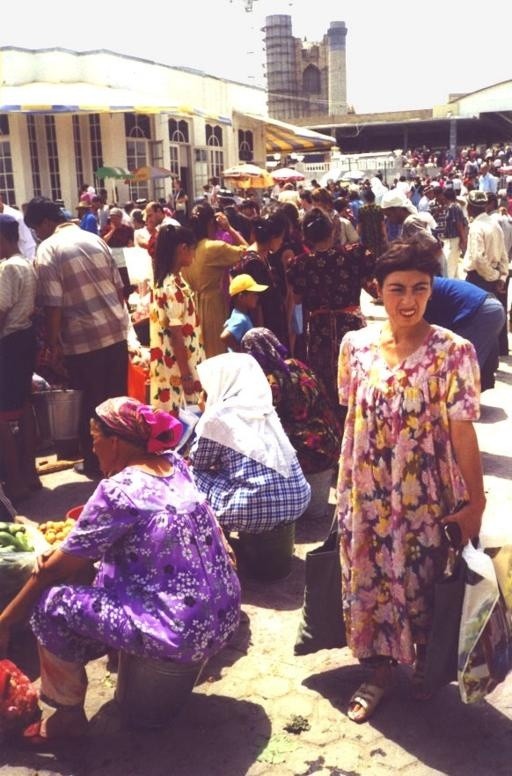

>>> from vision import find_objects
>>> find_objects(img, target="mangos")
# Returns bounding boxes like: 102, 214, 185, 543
0, 522, 35, 552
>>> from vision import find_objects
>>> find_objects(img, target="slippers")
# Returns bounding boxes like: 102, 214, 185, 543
346, 677, 390, 723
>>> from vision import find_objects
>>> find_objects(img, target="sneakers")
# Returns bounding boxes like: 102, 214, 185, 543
15, 718, 83, 751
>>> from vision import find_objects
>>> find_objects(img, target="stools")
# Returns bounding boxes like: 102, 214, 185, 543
233, 521, 294, 585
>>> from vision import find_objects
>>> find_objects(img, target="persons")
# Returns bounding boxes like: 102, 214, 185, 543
185, 351, 313, 537
0, 140, 511, 499
331, 236, 486, 727
0, 393, 246, 755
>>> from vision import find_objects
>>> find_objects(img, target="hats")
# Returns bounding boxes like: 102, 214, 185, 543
227, 273, 270, 297
486, 192, 499, 201
466, 189, 489, 208
422, 185, 434, 195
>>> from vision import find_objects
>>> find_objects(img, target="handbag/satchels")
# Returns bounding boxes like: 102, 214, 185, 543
421, 538, 481, 692
451, 536, 512, 706
291, 506, 352, 659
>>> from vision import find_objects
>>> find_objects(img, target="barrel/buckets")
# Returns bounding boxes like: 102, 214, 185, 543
238, 521, 296, 581
29, 389, 82, 440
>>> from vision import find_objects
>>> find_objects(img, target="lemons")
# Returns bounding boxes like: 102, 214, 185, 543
39, 519, 74, 544
